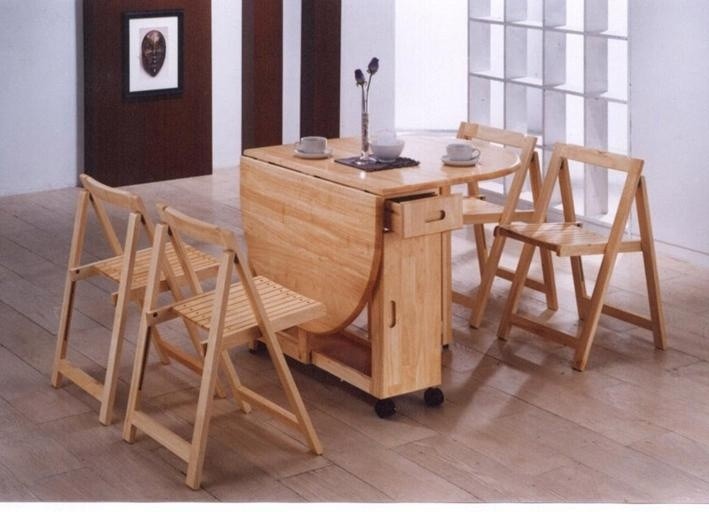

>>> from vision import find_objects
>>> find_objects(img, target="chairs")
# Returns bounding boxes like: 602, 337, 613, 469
496, 141, 668, 372
48, 172, 228, 428
120, 197, 326, 492
452, 121, 560, 330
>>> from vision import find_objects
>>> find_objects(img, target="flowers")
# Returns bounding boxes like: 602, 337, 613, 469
354, 57, 380, 150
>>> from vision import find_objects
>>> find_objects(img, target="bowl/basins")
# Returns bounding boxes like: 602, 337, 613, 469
370, 138, 404, 163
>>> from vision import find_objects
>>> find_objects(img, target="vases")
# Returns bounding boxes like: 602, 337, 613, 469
356, 112, 375, 164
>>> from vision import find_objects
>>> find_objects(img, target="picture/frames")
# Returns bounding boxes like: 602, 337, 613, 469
121, 9, 184, 96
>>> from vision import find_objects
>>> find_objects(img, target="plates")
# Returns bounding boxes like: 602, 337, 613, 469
441, 155, 478, 167
294, 149, 334, 159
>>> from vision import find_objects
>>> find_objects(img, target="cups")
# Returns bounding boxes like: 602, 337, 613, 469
446, 143, 480, 160
296, 136, 327, 153
